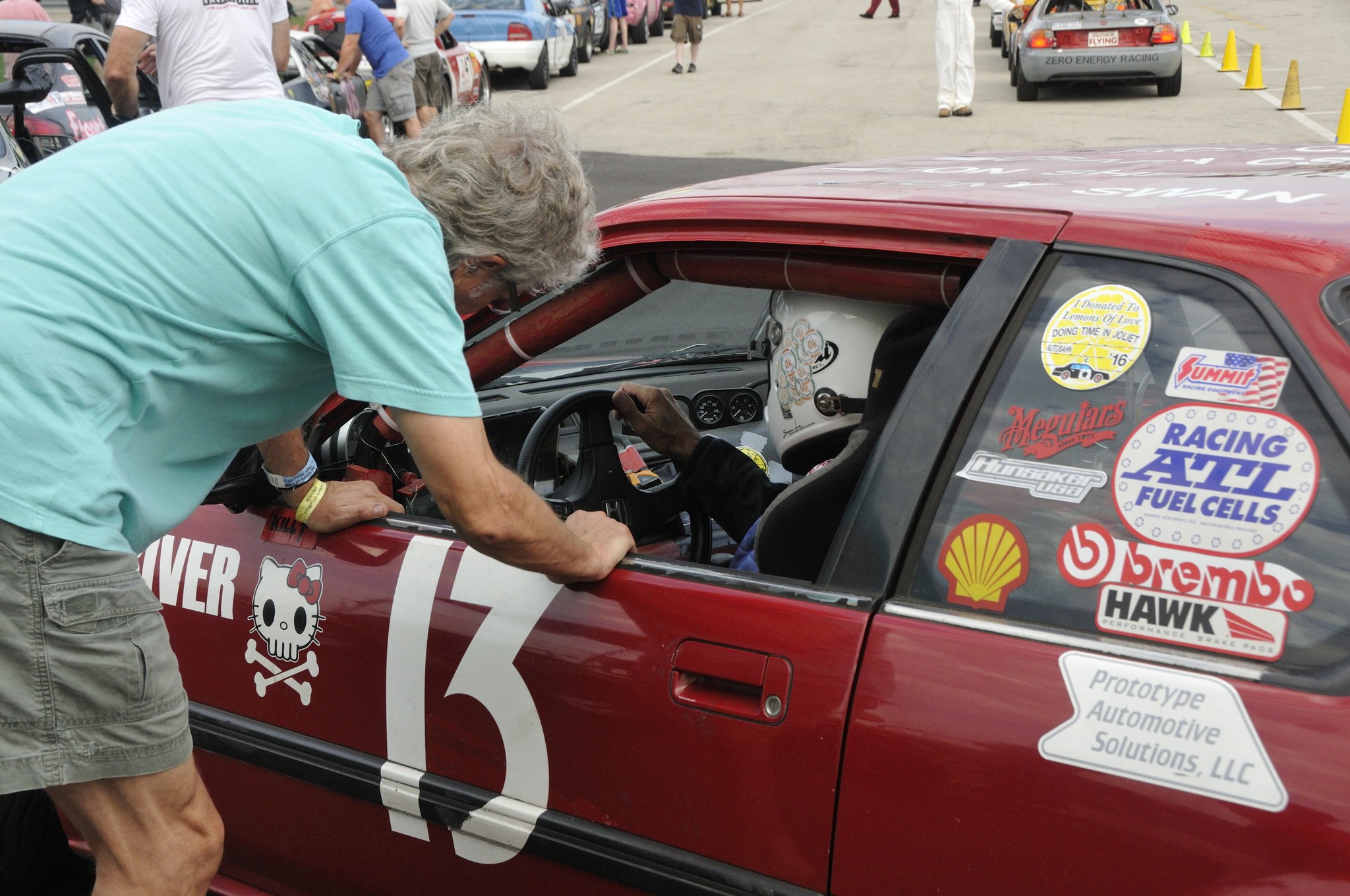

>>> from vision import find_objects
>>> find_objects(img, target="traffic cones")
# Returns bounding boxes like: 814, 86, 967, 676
1276, 60, 1306, 109
1240, 44, 1268, 90
1181, 20, 1193, 44
1334, 87, 1350, 144
1217, 30, 1242, 72
1196, 32, 1216, 58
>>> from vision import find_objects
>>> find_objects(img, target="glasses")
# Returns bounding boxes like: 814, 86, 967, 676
488, 280, 521, 314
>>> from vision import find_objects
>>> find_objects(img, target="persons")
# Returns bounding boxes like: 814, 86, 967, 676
67, 0, 121, 36
670, 0, 704, 73
326, 0, 423, 147
720, 0, 744, 17
0, 0, 52, 81
287, 0, 297, 21
612, 288, 914, 576
393, 0, 456, 128
304, 0, 336, 22
108, 0, 291, 123
600, 0, 630, 55
0, 94, 641, 896
935, 0, 1026, 117
860, 0, 900, 19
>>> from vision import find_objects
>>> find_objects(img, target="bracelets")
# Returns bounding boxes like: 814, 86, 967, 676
261, 448, 319, 492
113, 110, 139, 122
294, 478, 326, 525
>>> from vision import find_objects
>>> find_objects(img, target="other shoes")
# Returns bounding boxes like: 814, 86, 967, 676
688, 64, 696, 72
616, 49, 628, 53
888, 14, 899, 18
722, 13, 732, 17
860, 14, 873, 18
601, 50, 615, 54
938, 109, 950, 116
952, 107, 972, 115
672, 64, 682, 72
738, 13, 743, 16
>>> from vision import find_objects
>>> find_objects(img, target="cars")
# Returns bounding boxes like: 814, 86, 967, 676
1010, 0, 1183, 101
972, 0, 1144, 58
0, 18, 163, 179
551, 0, 609, 63
442, 1, 578, 90
131, 145, 1350, 896
280, 30, 396, 153
615, 0, 762, 44
302, 10, 493, 138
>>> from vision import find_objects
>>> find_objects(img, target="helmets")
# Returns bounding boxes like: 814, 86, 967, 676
747, 290, 940, 475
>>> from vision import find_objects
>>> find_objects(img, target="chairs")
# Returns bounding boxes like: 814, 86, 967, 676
758, 303, 946, 584
